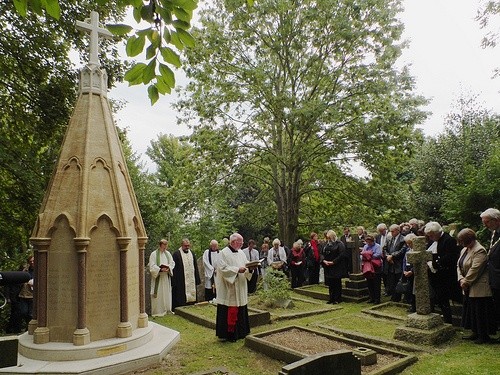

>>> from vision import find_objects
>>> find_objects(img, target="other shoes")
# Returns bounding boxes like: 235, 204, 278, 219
327, 300, 340, 304
382, 292, 389, 296
475, 336, 490, 344
462, 334, 475, 340
366, 299, 381, 305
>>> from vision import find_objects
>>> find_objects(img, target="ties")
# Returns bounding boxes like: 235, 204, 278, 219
249, 250, 251, 261
390, 238, 394, 251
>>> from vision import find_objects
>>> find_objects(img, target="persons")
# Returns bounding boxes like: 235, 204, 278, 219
362, 235, 383, 304
399, 218, 435, 313
424, 221, 463, 325
356, 226, 367, 241
304, 232, 320, 285
457, 228, 493, 344
203, 240, 221, 304
0, 256, 34, 334
242, 239, 259, 296
321, 230, 348, 304
375, 223, 391, 247
216, 233, 266, 342
172, 239, 202, 306
290, 239, 306, 288
382, 224, 407, 302
149, 239, 175, 317
340, 227, 350, 245
260, 237, 290, 292
261, 236, 270, 257
480, 208, 500, 344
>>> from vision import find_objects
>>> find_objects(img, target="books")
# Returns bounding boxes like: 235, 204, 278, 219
245, 257, 265, 267
159, 264, 169, 270
